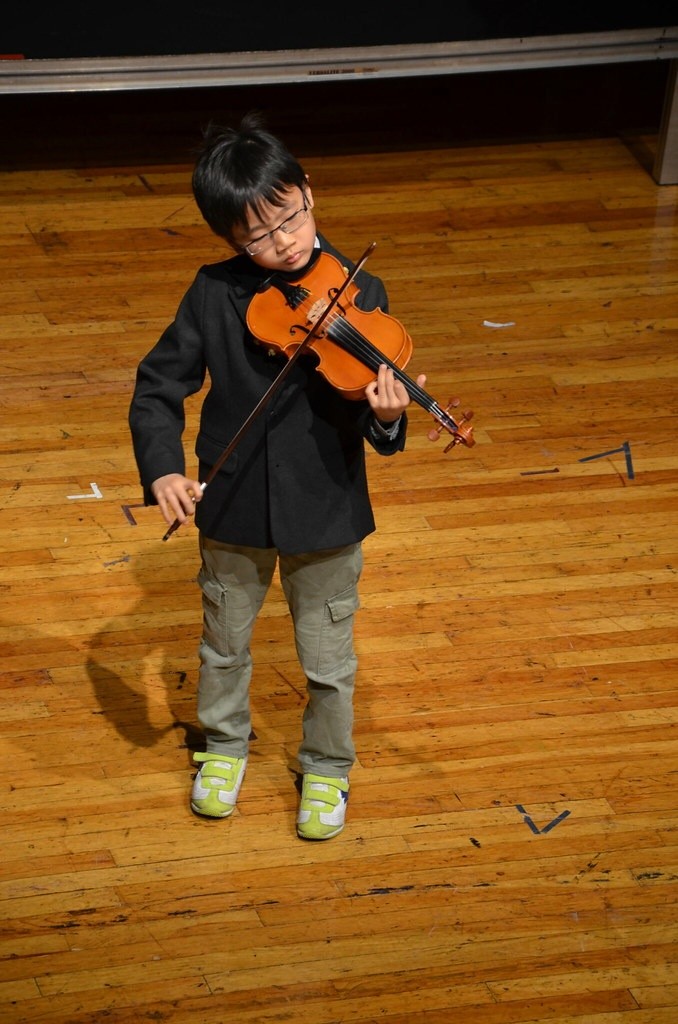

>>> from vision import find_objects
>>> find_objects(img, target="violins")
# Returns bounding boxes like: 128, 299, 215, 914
243, 251, 478, 454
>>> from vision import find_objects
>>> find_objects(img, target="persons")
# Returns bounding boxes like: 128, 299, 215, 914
129, 124, 427, 840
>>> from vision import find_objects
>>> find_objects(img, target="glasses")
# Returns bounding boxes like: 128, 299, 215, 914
230, 183, 310, 256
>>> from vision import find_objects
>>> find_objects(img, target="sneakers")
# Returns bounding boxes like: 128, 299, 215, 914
296, 773, 350, 839
190, 752, 248, 817
